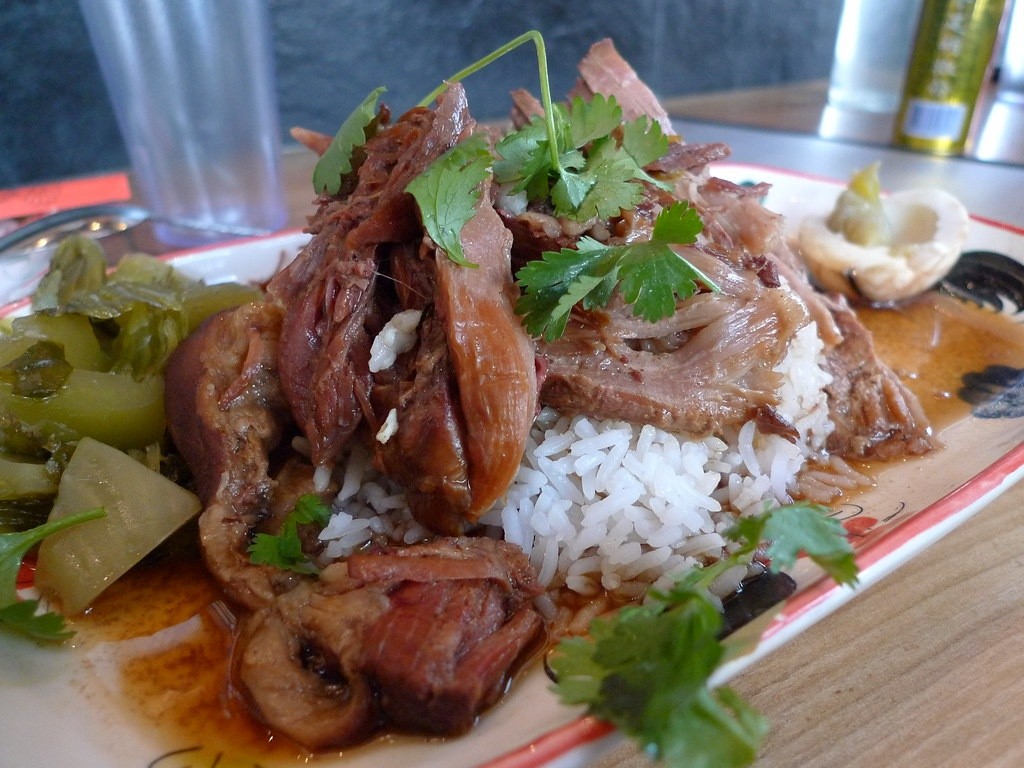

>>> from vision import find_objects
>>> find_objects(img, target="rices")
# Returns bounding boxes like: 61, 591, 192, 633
290, 308, 837, 632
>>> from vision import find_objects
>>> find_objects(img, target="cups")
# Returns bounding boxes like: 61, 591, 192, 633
77, 0, 287, 252
828, 0, 923, 115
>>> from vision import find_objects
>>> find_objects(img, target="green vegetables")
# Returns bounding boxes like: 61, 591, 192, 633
0, 29, 863, 767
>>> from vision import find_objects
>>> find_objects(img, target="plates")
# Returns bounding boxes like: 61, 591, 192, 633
0, 157, 1024, 768
